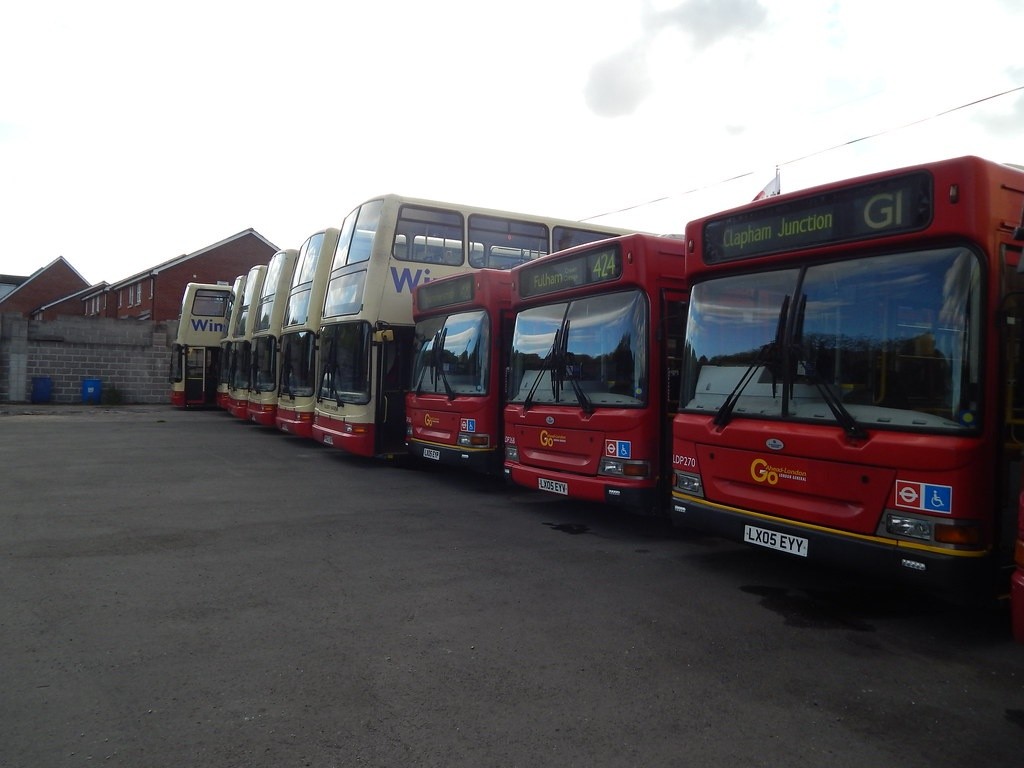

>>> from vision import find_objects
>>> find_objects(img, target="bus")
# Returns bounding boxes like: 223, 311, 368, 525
311, 196, 625, 462
503, 234, 685, 520
403, 267, 513, 479
274, 227, 339, 437
167, 250, 298, 427
667, 155, 1023, 592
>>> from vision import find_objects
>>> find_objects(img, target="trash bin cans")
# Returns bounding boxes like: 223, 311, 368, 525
81, 378, 101, 404
31, 376, 51, 403
